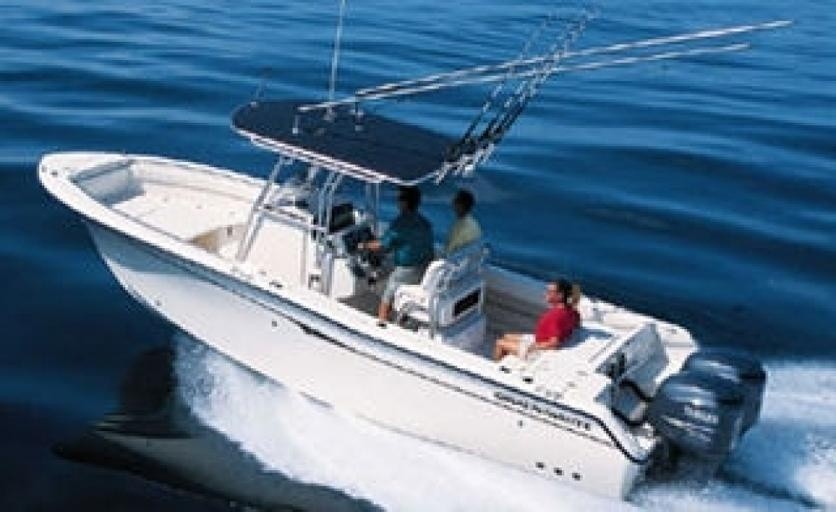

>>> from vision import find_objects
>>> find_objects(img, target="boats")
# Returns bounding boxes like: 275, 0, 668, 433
38, 0, 796, 499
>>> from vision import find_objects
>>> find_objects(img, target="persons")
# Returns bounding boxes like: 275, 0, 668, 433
491, 275, 583, 363
442, 185, 482, 252
353, 185, 436, 322
